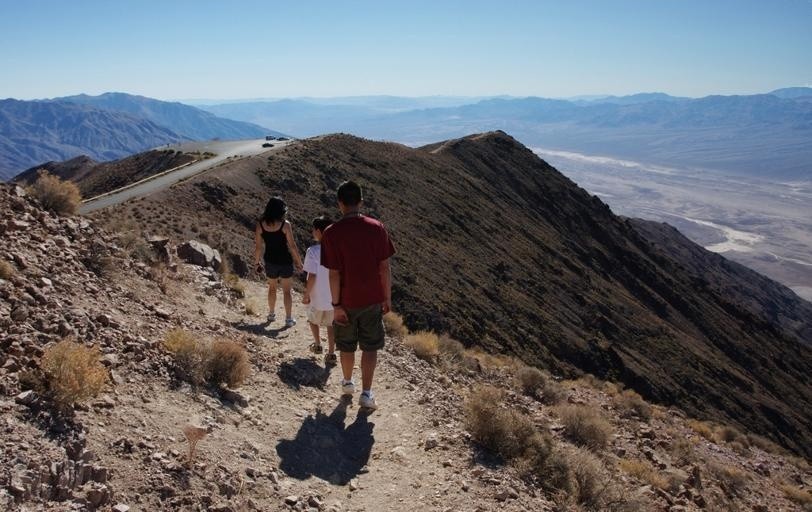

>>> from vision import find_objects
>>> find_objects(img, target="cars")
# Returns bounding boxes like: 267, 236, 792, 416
276, 136, 289, 140
263, 143, 274, 147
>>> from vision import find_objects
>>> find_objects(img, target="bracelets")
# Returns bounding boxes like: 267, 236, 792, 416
255, 262, 260, 266
331, 300, 340, 308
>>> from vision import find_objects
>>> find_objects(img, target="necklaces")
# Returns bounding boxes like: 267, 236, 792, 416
343, 211, 361, 217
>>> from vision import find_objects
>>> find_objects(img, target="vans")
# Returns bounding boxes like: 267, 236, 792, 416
266, 136, 275, 140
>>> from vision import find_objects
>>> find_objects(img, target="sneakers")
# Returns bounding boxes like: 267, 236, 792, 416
267, 314, 275, 321
285, 319, 296, 327
325, 353, 337, 364
310, 344, 323, 353
342, 379, 354, 392
360, 392, 377, 410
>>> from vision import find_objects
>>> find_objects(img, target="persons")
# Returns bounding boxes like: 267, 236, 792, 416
320, 180, 397, 411
300, 214, 341, 368
251, 196, 303, 328
